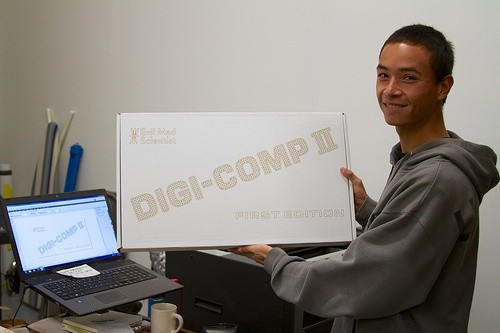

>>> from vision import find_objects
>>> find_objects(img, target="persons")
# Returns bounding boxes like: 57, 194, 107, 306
227, 23, 500, 333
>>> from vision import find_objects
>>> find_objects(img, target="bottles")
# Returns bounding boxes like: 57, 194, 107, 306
0, 165, 14, 199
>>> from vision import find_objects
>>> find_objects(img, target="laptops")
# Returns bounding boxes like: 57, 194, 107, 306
0, 189, 185, 317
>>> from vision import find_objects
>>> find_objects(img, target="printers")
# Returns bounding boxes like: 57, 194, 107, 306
166, 228, 362, 333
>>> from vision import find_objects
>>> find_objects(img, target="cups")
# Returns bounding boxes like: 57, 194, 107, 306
151, 303, 183, 333
